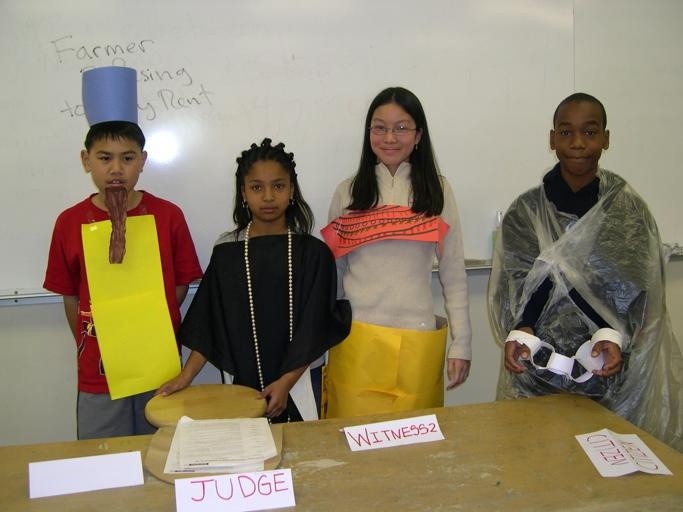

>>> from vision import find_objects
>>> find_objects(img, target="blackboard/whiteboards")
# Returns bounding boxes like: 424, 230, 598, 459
0, 0, 682, 300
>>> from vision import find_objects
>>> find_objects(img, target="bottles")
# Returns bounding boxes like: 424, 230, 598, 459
491, 210, 508, 252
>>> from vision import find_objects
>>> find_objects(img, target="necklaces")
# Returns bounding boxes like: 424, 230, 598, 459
244, 220, 295, 424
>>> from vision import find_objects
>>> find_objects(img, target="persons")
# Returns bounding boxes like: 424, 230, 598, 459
153, 137, 353, 423
322, 86, 473, 419
41, 121, 204, 440
487, 91, 683, 454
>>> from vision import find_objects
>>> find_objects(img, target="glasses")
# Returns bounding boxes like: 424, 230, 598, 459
370, 125, 416, 136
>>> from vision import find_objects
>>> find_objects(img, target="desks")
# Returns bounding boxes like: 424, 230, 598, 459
0, 391, 683, 512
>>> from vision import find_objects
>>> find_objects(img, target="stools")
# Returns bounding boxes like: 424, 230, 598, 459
145, 383, 267, 429
